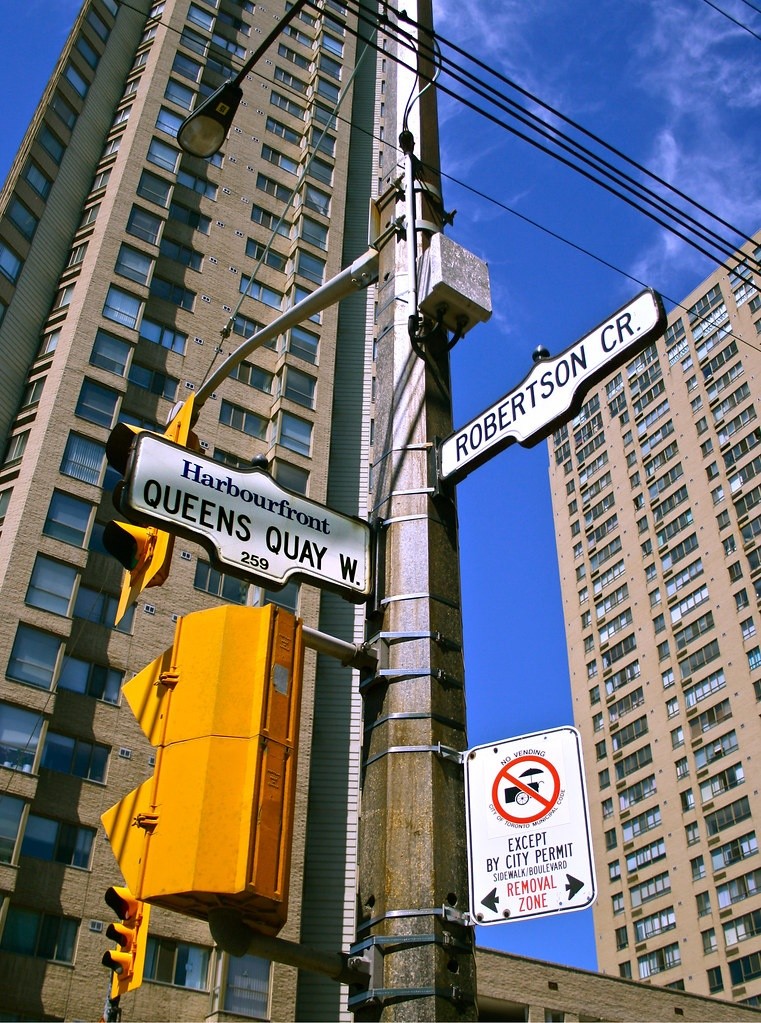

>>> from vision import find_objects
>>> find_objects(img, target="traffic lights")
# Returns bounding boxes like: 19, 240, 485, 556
100, 604, 304, 939
102, 391, 197, 626
100, 884, 152, 998
